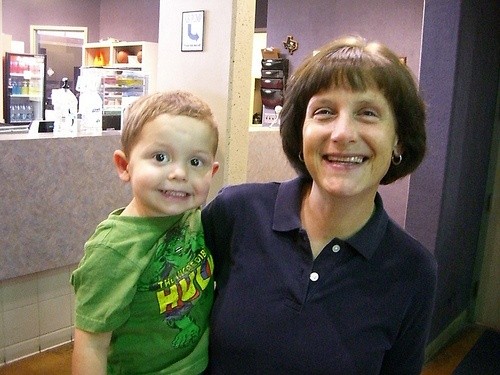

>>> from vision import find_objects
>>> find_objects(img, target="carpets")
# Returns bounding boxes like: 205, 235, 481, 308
450, 330, 500, 375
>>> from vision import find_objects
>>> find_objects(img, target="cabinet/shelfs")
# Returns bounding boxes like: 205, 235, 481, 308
98, 75, 146, 116
81, 42, 157, 71
260, 58, 289, 123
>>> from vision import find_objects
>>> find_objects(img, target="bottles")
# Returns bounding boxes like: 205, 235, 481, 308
10, 104, 32, 122
12, 80, 32, 95
53, 78, 78, 136
79, 82, 102, 135
94, 51, 100, 67
99, 51, 105, 66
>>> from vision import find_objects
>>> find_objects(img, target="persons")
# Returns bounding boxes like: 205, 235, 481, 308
201, 34, 438, 375
69, 90, 220, 375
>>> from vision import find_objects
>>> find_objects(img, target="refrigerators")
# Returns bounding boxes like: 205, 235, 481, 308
3, 53, 46, 125
